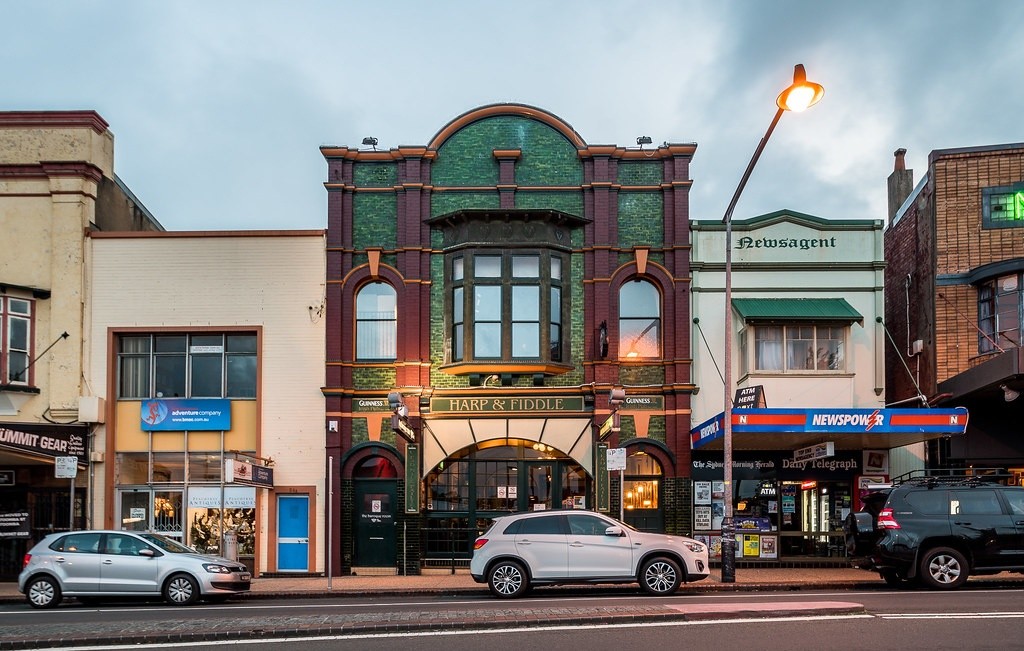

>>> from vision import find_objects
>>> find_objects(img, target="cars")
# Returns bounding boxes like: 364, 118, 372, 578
17, 530, 252, 608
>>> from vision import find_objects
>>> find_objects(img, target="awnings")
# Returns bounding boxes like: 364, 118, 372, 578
731, 297, 864, 327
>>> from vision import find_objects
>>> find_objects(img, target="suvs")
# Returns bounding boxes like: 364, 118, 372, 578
841, 467, 1024, 590
470, 508, 710, 600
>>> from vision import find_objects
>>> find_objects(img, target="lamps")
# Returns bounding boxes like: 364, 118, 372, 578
608, 389, 625, 405
388, 391, 402, 408
1001, 384, 1019, 401
637, 136, 652, 151
362, 137, 378, 152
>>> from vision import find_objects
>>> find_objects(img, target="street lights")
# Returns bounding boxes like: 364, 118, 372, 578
721, 63, 825, 583
